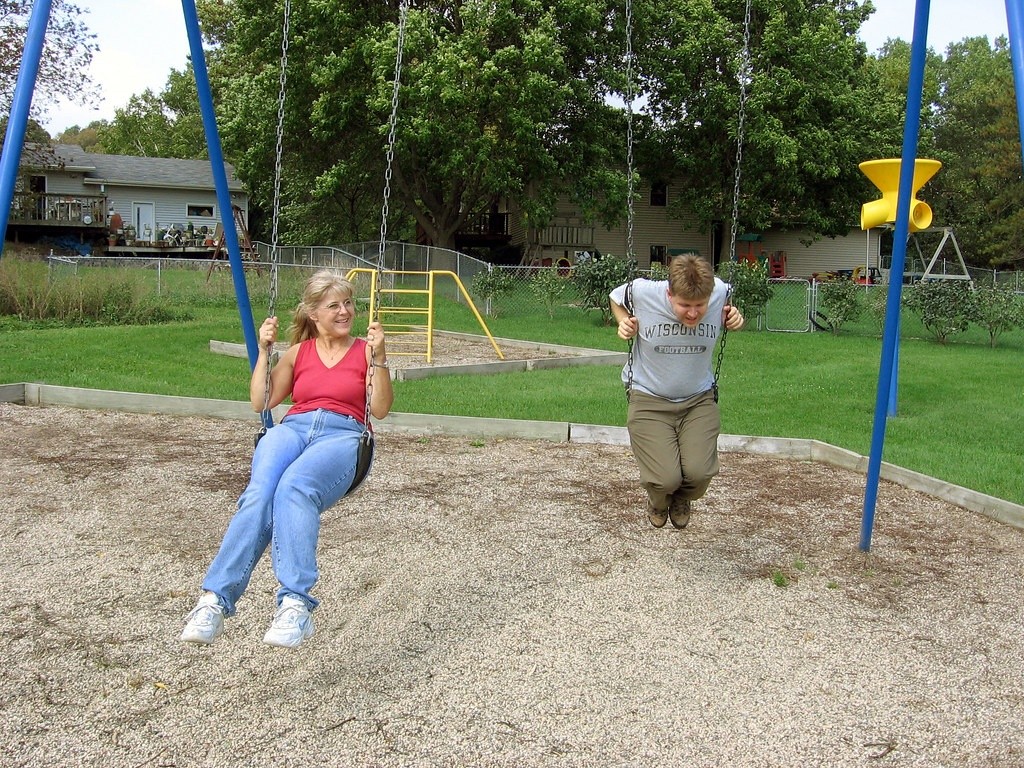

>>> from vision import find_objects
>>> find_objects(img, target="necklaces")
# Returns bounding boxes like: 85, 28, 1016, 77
316, 337, 351, 361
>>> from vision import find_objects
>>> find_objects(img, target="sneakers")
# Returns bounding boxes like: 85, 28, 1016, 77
179, 594, 224, 644
262, 596, 314, 648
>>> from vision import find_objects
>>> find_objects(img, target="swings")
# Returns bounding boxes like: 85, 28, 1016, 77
624, 0, 751, 405
252, 0, 410, 497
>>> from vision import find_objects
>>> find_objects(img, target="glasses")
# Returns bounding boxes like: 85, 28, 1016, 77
315, 299, 356, 312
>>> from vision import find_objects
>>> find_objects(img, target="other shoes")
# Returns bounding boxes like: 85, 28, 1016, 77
647, 496, 691, 529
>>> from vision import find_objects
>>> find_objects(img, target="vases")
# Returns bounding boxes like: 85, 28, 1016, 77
205, 240, 215, 246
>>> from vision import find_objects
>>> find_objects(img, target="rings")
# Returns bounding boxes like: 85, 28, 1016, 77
371, 334, 375, 342
266, 331, 269, 335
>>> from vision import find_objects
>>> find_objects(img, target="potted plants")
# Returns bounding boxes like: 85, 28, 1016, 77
108, 237, 117, 246
123, 224, 136, 246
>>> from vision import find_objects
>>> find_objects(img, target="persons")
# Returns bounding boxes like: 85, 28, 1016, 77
608, 252, 744, 530
179, 267, 394, 649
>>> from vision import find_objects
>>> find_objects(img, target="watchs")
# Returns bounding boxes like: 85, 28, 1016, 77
372, 359, 390, 369
